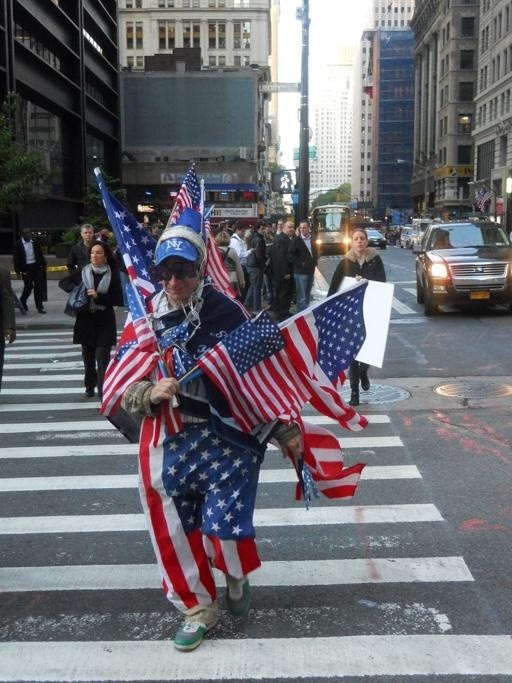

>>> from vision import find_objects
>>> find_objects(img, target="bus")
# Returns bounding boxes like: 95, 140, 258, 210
308, 202, 354, 255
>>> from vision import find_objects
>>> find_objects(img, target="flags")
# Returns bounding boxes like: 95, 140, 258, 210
169, 163, 235, 297
96, 173, 161, 415
476, 187, 492, 212
197, 280, 369, 501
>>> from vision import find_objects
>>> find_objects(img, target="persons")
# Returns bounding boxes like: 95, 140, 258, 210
326, 228, 387, 403
436, 236, 448, 248
101, 239, 303, 654
385, 229, 401, 245
66, 212, 162, 398
214, 219, 318, 321
1, 228, 47, 372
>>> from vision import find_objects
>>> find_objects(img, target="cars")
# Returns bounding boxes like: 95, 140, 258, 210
352, 211, 443, 251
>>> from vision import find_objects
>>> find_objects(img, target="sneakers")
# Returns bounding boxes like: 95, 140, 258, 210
225, 575, 250, 617
173, 603, 220, 649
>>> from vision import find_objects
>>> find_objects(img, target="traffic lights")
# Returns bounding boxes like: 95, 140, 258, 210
271, 169, 292, 193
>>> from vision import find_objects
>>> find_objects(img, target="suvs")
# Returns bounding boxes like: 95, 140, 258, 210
412, 216, 512, 314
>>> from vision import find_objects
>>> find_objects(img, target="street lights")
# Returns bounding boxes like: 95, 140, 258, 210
466, 180, 499, 222
396, 158, 428, 216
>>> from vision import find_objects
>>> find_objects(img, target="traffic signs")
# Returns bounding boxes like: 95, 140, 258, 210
255, 81, 301, 91
291, 145, 318, 160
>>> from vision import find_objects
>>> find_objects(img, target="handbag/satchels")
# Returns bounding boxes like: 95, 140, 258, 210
68, 282, 90, 314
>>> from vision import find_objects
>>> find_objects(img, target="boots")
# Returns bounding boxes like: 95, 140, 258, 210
349, 378, 359, 406
360, 368, 369, 390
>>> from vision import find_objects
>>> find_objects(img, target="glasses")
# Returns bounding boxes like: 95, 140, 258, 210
156, 266, 192, 279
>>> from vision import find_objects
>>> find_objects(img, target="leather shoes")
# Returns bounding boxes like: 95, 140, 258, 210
38, 308, 47, 314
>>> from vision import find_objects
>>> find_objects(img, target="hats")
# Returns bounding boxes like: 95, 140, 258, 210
154, 225, 207, 266
